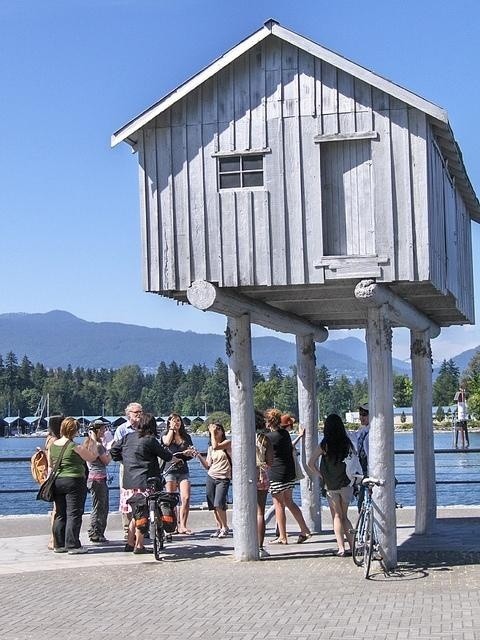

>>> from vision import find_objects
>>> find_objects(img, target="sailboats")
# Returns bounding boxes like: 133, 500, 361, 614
14, 393, 116, 438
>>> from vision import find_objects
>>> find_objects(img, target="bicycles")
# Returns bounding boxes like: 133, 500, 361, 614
143, 458, 188, 559
349, 474, 385, 579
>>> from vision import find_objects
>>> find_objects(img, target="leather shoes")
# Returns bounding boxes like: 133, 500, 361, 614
334, 550, 346, 557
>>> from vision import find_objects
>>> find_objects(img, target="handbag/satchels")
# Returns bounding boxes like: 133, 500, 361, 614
36, 473, 57, 502
343, 454, 363, 481
256, 434, 267, 468
126, 493, 150, 534
157, 492, 179, 532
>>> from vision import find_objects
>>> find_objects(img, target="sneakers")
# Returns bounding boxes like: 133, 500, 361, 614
269, 538, 288, 545
125, 543, 134, 552
91, 536, 109, 545
133, 544, 153, 554
69, 546, 88, 553
294, 533, 312, 544
259, 550, 270, 558
54, 546, 67, 553
210, 527, 229, 537
177, 526, 195, 535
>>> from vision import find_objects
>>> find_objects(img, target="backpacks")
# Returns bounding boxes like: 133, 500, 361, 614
31, 436, 52, 483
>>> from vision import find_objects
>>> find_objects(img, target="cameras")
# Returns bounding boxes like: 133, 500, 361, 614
84, 426, 96, 438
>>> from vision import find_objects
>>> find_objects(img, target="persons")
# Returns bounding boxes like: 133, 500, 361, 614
356, 402, 369, 478
47, 416, 98, 555
264, 411, 305, 538
191, 422, 233, 539
160, 411, 197, 535
43, 415, 65, 551
305, 413, 358, 557
109, 411, 173, 555
112, 401, 144, 542
261, 407, 312, 544
255, 410, 273, 559
82, 418, 114, 546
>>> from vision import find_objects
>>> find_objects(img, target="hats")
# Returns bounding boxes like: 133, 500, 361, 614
94, 420, 109, 426
212, 418, 225, 427
356, 402, 368, 410
280, 414, 295, 427
